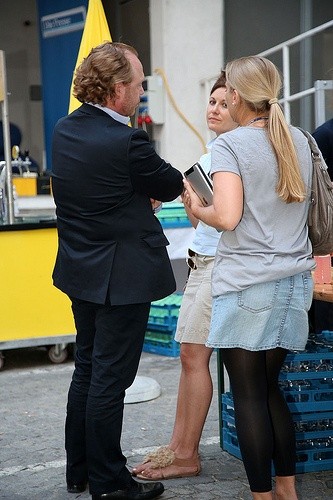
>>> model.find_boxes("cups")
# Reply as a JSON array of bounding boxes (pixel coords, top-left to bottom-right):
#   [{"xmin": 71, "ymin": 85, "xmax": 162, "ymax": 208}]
[{"xmin": 314, "ymin": 256, "xmax": 333, "ymax": 292}]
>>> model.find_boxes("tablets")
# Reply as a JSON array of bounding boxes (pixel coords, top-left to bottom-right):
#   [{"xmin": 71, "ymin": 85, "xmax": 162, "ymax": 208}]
[{"xmin": 184, "ymin": 162, "xmax": 224, "ymax": 233}]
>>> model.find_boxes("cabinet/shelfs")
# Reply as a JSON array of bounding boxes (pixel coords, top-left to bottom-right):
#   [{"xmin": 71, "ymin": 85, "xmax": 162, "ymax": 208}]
[{"xmin": 0, "ymin": 193, "xmax": 81, "ymax": 350}]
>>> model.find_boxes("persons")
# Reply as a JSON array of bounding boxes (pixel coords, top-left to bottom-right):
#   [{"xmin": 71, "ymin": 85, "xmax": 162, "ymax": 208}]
[
  {"xmin": 130, "ymin": 69, "xmax": 238, "ymax": 481},
  {"xmin": 48, "ymin": 40, "xmax": 182, "ymax": 500},
  {"xmin": 181, "ymin": 55, "xmax": 329, "ymax": 500},
  {"xmin": 0, "ymin": 119, "xmax": 22, "ymax": 161},
  {"xmin": 311, "ymin": 118, "xmax": 333, "ymax": 182}
]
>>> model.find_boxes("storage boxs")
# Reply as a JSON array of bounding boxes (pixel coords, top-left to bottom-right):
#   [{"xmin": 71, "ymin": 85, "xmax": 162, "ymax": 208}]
[
  {"xmin": 142, "ymin": 290, "xmax": 185, "ymax": 358},
  {"xmin": 217, "ymin": 331, "xmax": 333, "ymax": 473},
  {"xmin": 12, "ymin": 174, "xmax": 37, "ymax": 197}
]
